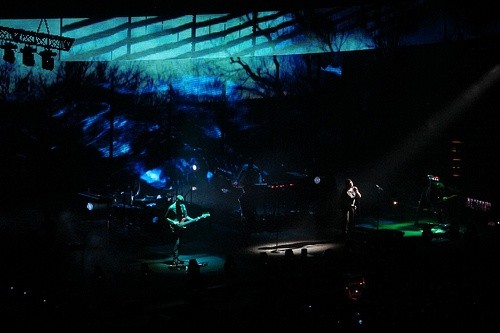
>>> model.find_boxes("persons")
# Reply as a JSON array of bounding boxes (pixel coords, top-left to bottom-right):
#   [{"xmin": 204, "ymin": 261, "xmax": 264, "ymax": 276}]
[
  {"xmin": 163, "ymin": 194, "xmax": 199, "ymax": 266},
  {"xmin": 130, "ymin": 178, "xmax": 146, "ymax": 227},
  {"xmin": 426, "ymin": 176, "xmax": 448, "ymax": 225},
  {"xmin": 237, "ymin": 184, "xmax": 257, "ymax": 244},
  {"xmin": 341, "ymin": 178, "xmax": 362, "ymax": 241}
]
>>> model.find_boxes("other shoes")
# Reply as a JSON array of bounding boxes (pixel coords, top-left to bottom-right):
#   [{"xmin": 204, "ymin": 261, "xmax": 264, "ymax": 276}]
[{"xmin": 172, "ymin": 258, "xmax": 184, "ymax": 264}]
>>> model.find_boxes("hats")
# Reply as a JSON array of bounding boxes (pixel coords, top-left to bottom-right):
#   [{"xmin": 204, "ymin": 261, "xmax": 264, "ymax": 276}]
[{"xmin": 176, "ymin": 194, "xmax": 184, "ymax": 203}]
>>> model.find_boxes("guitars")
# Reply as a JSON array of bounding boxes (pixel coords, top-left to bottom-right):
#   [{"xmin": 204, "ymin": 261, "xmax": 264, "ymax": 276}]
[{"xmin": 170, "ymin": 212, "xmax": 211, "ymax": 232}]
